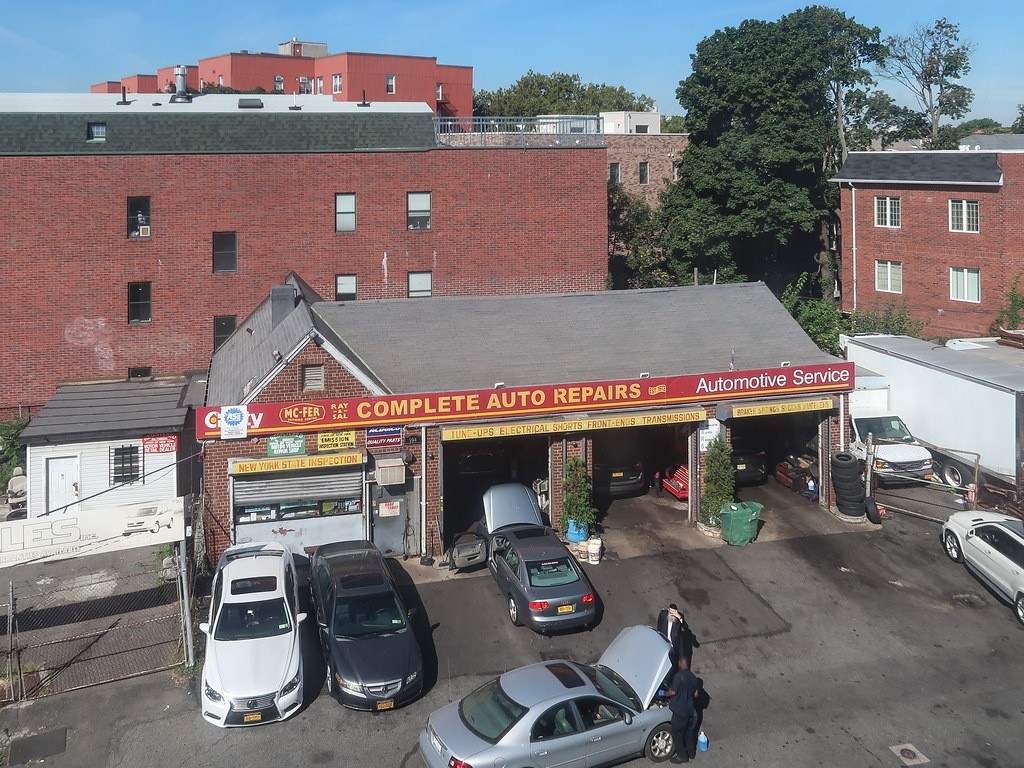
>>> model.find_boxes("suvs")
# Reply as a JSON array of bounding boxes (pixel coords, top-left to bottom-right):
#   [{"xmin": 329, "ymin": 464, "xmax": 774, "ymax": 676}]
[{"xmin": 848, "ymin": 410, "xmax": 934, "ymax": 489}]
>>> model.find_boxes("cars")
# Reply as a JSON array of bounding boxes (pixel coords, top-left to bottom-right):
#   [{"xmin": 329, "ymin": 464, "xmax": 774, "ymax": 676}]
[
  {"xmin": 307, "ymin": 539, "xmax": 441, "ymax": 712},
  {"xmin": 198, "ymin": 540, "xmax": 307, "ymax": 729},
  {"xmin": 418, "ymin": 626, "xmax": 674, "ymax": 768},
  {"xmin": 731, "ymin": 448, "xmax": 768, "ymax": 488},
  {"xmin": 593, "ymin": 446, "xmax": 650, "ymax": 499},
  {"xmin": 449, "ymin": 482, "xmax": 596, "ymax": 635},
  {"xmin": 942, "ymin": 510, "xmax": 1024, "ymax": 626}
]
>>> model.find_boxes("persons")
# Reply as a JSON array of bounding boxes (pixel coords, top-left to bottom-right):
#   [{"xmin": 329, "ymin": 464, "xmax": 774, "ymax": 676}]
[
  {"xmin": 652, "ymin": 463, "xmax": 679, "ymax": 498},
  {"xmin": 657, "ymin": 604, "xmax": 688, "ymax": 657},
  {"xmin": 664, "ymin": 657, "xmax": 700, "ymax": 763}
]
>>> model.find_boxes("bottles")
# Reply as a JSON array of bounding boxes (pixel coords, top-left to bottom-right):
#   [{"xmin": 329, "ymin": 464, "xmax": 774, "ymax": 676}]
[{"xmin": 698, "ymin": 731, "xmax": 708, "ymax": 752}]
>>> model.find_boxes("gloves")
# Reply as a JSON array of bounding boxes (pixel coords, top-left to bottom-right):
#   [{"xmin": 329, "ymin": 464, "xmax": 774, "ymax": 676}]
[{"xmin": 658, "ymin": 690, "xmax": 665, "ymax": 696}]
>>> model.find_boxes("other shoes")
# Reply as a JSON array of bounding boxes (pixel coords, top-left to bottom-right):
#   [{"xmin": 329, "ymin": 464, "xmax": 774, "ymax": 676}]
[
  {"xmin": 687, "ymin": 752, "xmax": 696, "ymax": 760},
  {"xmin": 670, "ymin": 755, "xmax": 688, "ymax": 764}
]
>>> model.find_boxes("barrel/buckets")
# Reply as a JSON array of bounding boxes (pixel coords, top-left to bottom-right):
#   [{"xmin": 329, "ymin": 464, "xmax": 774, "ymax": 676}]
[{"xmin": 577, "ymin": 539, "xmax": 603, "ymax": 564}]
